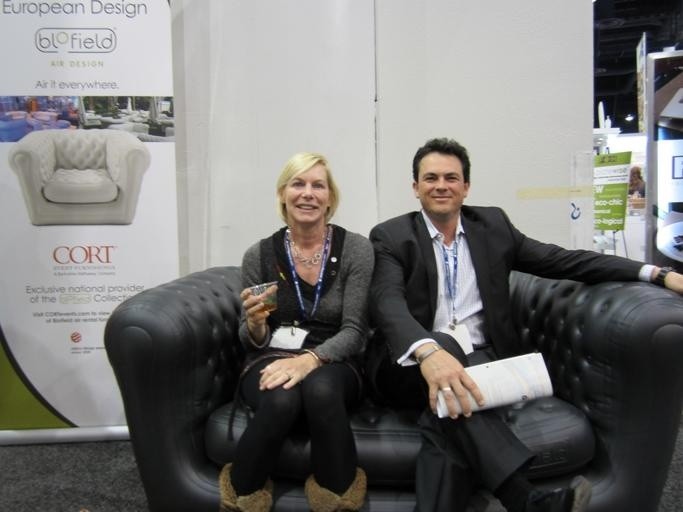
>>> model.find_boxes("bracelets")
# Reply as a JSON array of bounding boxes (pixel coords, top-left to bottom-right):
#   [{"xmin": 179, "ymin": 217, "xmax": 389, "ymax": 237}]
[
  {"xmin": 652, "ymin": 265, "xmax": 678, "ymax": 288},
  {"xmin": 299, "ymin": 344, "xmax": 322, "ymax": 370},
  {"xmin": 415, "ymin": 344, "xmax": 444, "ymax": 365}
]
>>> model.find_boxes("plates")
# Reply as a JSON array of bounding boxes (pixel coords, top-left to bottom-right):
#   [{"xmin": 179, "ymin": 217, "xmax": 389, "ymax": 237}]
[{"xmin": 656, "ymin": 219, "xmax": 682, "ymax": 262}]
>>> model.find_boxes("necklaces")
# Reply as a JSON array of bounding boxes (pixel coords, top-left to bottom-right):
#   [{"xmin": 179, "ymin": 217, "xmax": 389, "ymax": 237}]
[{"xmin": 283, "ymin": 222, "xmax": 329, "ymax": 270}]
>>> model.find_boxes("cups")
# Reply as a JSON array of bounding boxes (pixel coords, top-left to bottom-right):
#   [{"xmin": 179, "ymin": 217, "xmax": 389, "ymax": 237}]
[{"xmin": 251, "ymin": 280, "xmax": 280, "ymax": 313}]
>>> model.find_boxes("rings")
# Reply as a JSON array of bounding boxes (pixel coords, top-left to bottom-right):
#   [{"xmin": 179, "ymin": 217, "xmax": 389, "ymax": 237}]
[
  {"xmin": 263, "ymin": 364, "xmax": 273, "ymax": 375},
  {"xmin": 284, "ymin": 369, "xmax": 295, "ymax": 383},
  {"xmin": 442, "ymin": 385, "xmax": 452, "ymax": 397}
]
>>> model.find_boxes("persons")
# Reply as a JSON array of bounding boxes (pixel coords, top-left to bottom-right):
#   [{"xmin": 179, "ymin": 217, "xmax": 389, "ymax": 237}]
[
  {"xmin": 365, "ymin": 136, "xmax": 682, "ymax": 511},
  {"xmin": 217, "ymin": 149, "xmax": 379, "ymax": 511},
  {"xmin": 627, "ymin": 164, "xmax": 645, "ymax": 199}
]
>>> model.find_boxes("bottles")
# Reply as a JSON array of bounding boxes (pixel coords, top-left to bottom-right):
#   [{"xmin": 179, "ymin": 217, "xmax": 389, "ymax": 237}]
[{"xmin": 604, "ymin": 115, "xmax": 612, "ymax": 128}]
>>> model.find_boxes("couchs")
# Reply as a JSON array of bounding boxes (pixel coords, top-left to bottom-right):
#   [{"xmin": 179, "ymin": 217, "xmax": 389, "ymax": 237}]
[
  {"xmin": 7, "ymin": 125, "xmax": 150, "ymax": 227},
  {"xmin": 100, "ymin": 256, "xmax": 682, "ymax": 512}
]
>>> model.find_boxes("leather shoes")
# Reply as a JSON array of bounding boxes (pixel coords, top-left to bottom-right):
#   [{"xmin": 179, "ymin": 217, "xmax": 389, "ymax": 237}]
[{"xmin": 528, "ymin": 473, "xmax": 594, "ymax": 511}]
